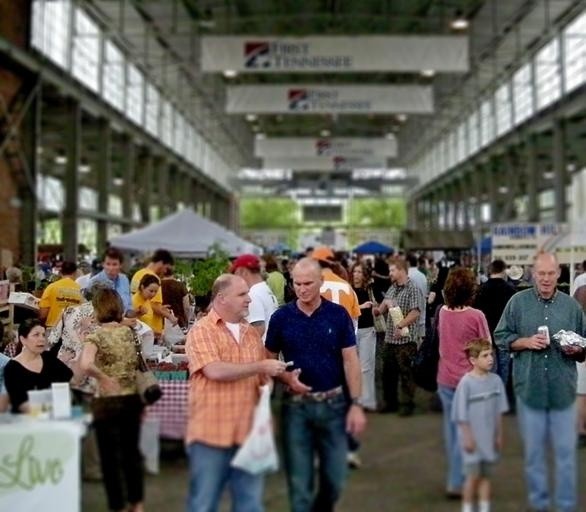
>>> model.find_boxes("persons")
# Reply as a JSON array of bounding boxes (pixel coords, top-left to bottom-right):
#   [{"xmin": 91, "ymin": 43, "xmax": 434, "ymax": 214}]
[
  {"xmin": 161, "ymin": 266, "xmax": 191, "ymax": 331},
  {"xmin": 307, "ymin": 245, "xmax": 361, "ymax": 323},
  {"xmin": 131, "ymin": 273, "xmax": 159, "ymax": 327},
  {"xmin": 226, "ymin": 253, "xmax": 278, "ymax": 346},
  {"xmin": 372, "ymin": 255, "xmax": 425, "ymax": 418},
  {"xmin": 428, "ymin": 267, "xmax": 449, "ymax": 327},
  {"xmin": 493, "ymin": 253, "xmax": 586, "ymax": 512},
  {"xmin": 473, "ymin": 258, "xmax": 517, "ymax": 416},
  {"xmin": 47, "ymin": 283, "xmax": 112, "ymax": 403},
  {"xmin": 89, "ymin": 248, "xmax": 147, "ymax": 319},
  {"xmin": 185, "ymin": 274, "xmax": 286, "ymax": 510},
  {"xmin": 0, "ymin": 321, "xmax": 12, "ymax": 413},
  {"xmin": 266, "ymin": 261, "xmax": 287, "ymax": 307},
  {"xmin": 406, "ymin": 254, "xmax": 427, "ymax": 348},
  {"xmin": 264, "ymin": 257, "xmax": 366, "ymax": 512},
  {"xmin": 436, "ymin": 269, "xmax": 492, "ymax": 500},
  {"xmin": 349, "ymin": 261, "xmax": 378, "ymax": 414},
  {"xmin": 129, "ymin": 248, "xmax": 180, "ymax": 344},
  {"xmin": 80, "ymin": 287, "xmax": 145, "ymax": 512},
  {"xmin": 5, "ymin": 260, "xmax": 26, "ymax": 298},
  {"xmin": 450, "ymin": 338, "xmax": 509, "ymax": 512},
  {"xmin": 39, "ymin": 261, "xmax": 83, "ymax": 327},
  {"xmin": 573, "ymin": 260, "xmax": 586, "ymax": 295},
  {"xmin": 573, "ymin": 287, "xmax": 586, "ymax": 450},
  {"xmin": 4, "ymin": 318, "xmax": 76, "ymax": 415}
]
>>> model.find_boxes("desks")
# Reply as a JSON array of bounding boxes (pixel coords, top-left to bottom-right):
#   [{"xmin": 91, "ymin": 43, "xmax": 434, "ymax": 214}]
[
  {"xmin": 144, "ymin": 379, "xmax": 190, "ymax": 440},
  {"xmin": 0, "ymin": 404, "xmax": 93, "ymax": 511}
]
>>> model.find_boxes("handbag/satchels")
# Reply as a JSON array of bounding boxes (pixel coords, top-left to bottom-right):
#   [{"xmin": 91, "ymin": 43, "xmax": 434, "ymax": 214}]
[{"xmin": 128, "ymin": 325, "xmax": 164, "ymax": 407}]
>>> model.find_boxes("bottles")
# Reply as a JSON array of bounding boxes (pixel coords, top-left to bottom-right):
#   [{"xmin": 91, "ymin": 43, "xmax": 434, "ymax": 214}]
[{"xmin": 539, "ymin": 325, "xmax": 551, "ymax": 345}]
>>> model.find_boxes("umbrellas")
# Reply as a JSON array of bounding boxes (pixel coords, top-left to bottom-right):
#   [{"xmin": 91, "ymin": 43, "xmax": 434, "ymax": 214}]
[
  {"xmin": 352, "ymin": 241, "xmax": 395, "ymax": 255},
  {"xmin": 110, "ymin": 209, "xmax": 263, "ymax": 260}
]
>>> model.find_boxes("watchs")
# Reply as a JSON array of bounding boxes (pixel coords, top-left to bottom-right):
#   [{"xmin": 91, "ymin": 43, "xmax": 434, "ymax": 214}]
[{"xmin": 350, "ymin": 397, "xmax": 365, "ymax": 408}]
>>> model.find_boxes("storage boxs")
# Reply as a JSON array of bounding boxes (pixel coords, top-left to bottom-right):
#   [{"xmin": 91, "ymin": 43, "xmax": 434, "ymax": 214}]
[
  {"xmin": 171, "ymin": 345, "xmax": 185, "ymax": 353},
  {"xmin": 8, "ymin": 292, "xmax": 40, "ymax": 310},
  {"xmin": 171, "ymin": 353, "xmax": 187, "ymax": 366},
  {"xmin": 171, "ymin": 371, "xmax": 187, "ymax": 380},
  {"xmin": 155, "ymin": 371, "xmax": 170, "ymax": 380},
  {"xmin": 174, "ymin": 335, "xmax": 186, "ymax": 345}
]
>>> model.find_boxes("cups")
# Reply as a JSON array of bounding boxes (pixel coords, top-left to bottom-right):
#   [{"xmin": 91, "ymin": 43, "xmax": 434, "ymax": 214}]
[
  {"xmin": 52, "ymin": 383, "xmax": 70, "ymax": 418},
  {"xmin": 27, "ymin": 390, "xmax": 45, "ymax": 417}
]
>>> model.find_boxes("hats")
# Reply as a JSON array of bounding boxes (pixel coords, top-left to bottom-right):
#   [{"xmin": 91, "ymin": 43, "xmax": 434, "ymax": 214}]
[
  {"xmin": 505, "ymin": 266, "xmax": 523, "ymax": 279},
  {"xmin": 312, "ymin": 247, "xmax": 335, "ymax": 263},
  {"xmin": 230, "ymin": 255, "xmax": 260, "ymax": 272}
]
